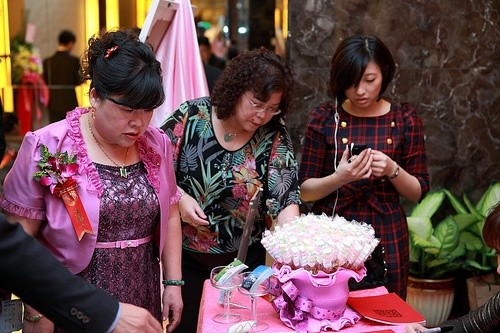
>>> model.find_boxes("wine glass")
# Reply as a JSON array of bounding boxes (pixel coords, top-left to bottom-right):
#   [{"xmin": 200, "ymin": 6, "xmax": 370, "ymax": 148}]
[
  {"xmin": 209, "ymin": 265, "xmax": 243, "ymax": 323},
  {"xmin": 234, "ymin": 272, "xmax": 271, "ymax": 332}
]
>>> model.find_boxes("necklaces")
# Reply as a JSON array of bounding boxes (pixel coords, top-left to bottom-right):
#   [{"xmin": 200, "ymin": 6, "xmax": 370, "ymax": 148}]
[
  {"xmin": 86, "ymin": 111, "xmax": 131, "ymax": 179},
  {"xmin": 219, "ymin": 117, "xmax": 248, "ymax": 142}
]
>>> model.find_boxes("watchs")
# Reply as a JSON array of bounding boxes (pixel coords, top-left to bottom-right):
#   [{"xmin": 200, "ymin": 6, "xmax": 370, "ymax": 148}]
[{"xmin": 388, "ymin": 164, "xmax": 401, "ymax": 180}]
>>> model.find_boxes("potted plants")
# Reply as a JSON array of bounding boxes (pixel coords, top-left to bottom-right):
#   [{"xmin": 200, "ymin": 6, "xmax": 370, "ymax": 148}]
[{"xmin": 406, "ymin": 183, "xmax": 500, "ymax": 328}]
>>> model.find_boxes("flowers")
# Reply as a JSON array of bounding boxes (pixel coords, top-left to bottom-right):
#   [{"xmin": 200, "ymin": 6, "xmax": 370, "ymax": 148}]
[{"xmin": 32, "ymin": 144, "xmax": 80, "ymax": 195}]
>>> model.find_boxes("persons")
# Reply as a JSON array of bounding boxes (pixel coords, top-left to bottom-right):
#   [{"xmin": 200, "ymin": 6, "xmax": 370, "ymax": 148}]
[
  {"xmin": 42, "ymin": 28, "xmax": 86, "ymax": 126},
  {"xmin": 0, "ymin": 210, "xmax": 164, "ymax": 333},
  {"xmin": 196, "ymin": 36, "xmax": 227, "ymax": 97},
  {"xmin": 157, "ymin": 49, "xmax": 301, "ymax": 333},
  {"xmin": 0, "ymin": 28, "xmax": 186, "ymax": 333},
  {"xmin": 405, "ymin": 202, "xmax": 500, "ymax": 333},
  {"xmin": 299, "ymin": 34, "xmax": 430, "ymax": 308}
]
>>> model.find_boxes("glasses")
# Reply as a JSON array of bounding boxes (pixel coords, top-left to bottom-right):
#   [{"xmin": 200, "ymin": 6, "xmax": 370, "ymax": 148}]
[{"xmin": 244, "ymin": 89, "xmax": 281, "ymax": 115}]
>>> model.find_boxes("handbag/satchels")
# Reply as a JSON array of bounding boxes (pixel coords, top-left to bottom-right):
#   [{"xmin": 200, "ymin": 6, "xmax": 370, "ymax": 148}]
[{"xmin": 349, "ymin": 240, "xmax": 388, "ymax": 290}]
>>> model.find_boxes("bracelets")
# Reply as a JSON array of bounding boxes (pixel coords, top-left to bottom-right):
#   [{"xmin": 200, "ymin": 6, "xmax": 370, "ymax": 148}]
[
  {"xmin": 162, "ymin": 279, "xmax": 185, "ymax": 286},
  {"xmin": 24, "ymin": 312, "xmax": 44, "ymax": 321}
]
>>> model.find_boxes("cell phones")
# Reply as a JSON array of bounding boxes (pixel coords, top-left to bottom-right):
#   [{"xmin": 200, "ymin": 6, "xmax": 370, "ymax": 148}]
[{"xmin": 348, "ymin": 144, "xmax": 368, "ymax": 156}]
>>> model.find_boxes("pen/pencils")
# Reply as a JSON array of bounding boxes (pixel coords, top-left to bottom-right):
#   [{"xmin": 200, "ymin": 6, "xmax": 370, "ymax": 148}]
[{"xmin": 414, "ymin": 326, "xmax": 455, "ymax": 333}]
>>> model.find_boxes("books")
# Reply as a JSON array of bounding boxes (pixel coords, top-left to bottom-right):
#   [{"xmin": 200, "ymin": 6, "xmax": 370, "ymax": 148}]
[{"xmin": 346, "ymin": 293, "xmax": 427, "ymax": 327}]
